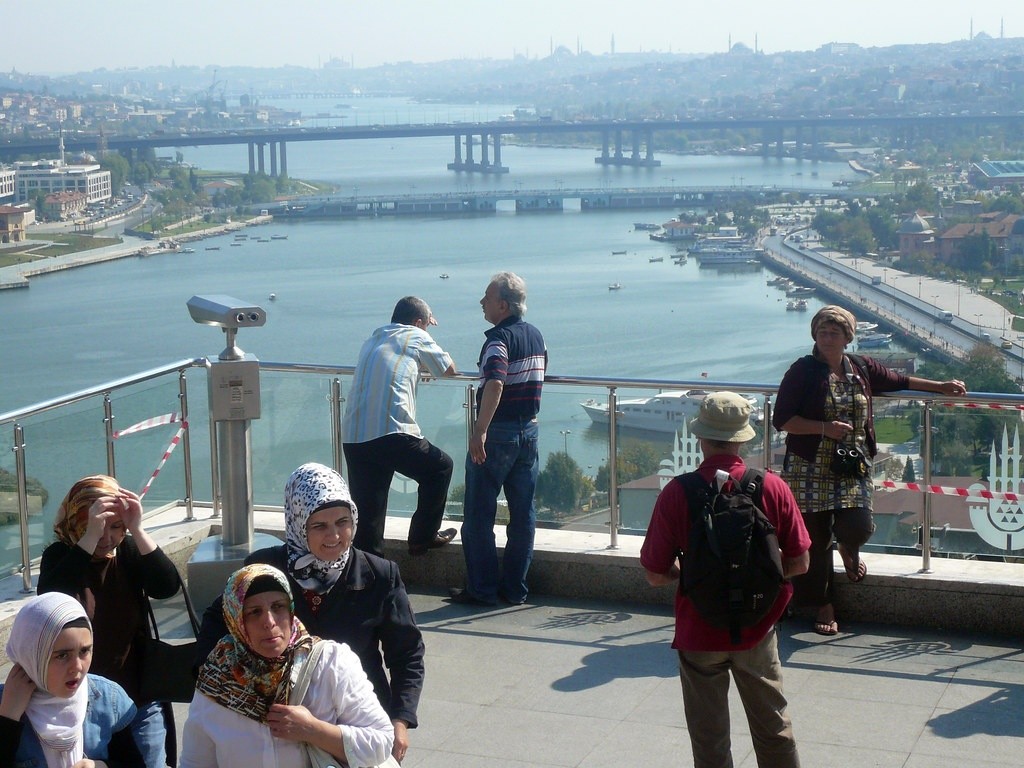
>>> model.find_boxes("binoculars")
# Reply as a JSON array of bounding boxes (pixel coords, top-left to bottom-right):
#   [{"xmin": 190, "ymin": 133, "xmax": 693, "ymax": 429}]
[
  {"xmin": 831, "ymin": 448, "xmax": 858, "ymax": 474},
  {"xmin": 187, "ymin": 294, "xmax": 267, "ymax": 328}
]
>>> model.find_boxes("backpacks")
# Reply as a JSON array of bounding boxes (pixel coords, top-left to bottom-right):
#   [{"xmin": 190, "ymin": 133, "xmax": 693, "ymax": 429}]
[{"xmin": 673, "ymin": 468, "xmax": 788, "ymax": 632}]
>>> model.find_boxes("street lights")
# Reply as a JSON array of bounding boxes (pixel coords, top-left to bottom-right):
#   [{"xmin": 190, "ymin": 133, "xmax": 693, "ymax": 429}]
[{"xmin": 559, "ymin": 430, "xmax": 571, "ymax": 459}]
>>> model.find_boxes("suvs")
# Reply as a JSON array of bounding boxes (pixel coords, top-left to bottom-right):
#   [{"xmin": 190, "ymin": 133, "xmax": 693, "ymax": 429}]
[{"xmin": 984, "ymin": 334, "xmax": 990, "ymax": 340}]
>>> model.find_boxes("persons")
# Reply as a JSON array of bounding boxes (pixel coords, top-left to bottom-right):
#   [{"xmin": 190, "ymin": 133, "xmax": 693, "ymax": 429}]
[
  {"xmin": 451, "ymin": 272, "xmax": 548, "ymax": 605},
  {"xmin": 37, "ymin": 474, "xmax": 181, "ymax": 768},
  {"xmin": 640, "ymin": 390, "xmax": 812, "ymax": 768},
  {"xmin": 200, "ymin": 462, "xmax": 425, "ymax": 766},
  {"xmin": 178, "ymin": 564, "xmax": 395, "ymax": 768},
  {"xmin": 0, "ymin": 592, "xmax": 148, "ymax": 768},
  {"xmin": 773, "ymin": 304, "xmax": 966, "ymax": 635},
  {"xmin": 341, "ymin": 296, "xmax": 457, "ymax": 557}
]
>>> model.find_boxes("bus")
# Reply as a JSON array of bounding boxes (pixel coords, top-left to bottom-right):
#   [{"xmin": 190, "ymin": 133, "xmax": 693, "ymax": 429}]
[{"xmin": 770, "ymin": 226, "xmax": 777, "ymax": 236}]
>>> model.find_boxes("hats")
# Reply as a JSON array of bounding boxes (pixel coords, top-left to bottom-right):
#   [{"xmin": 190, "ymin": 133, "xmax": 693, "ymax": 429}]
[{"xmin": 690, "ymin": 391, "xmax": 756, "ymax": 443}]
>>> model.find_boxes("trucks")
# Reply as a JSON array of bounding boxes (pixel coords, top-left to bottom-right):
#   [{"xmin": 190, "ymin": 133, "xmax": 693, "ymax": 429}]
[{"xmin": 872, "ymin": 276, "xmax": 881, "ymax": 284}]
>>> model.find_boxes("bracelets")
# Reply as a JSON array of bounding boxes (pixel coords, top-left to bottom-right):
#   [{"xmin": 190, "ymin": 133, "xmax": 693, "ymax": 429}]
[{"xmin": 821, "ymin": 421, "xmax": 825, "ymax": 439}]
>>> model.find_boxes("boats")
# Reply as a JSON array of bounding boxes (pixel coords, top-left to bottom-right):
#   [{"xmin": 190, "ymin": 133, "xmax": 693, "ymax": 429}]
[
  {"xmin": 857, "ymin": 330, "xmax": 892, "ymax": 347},
  {"xmin": 608, "ymin": 282, "xmax": 620, "ymax": 289},
  {"xmin": 634, "ymin": 223, "xmax": 661, "ymax": 229},
  {"xmin": 271, "ymin": 235, "xmax": 288, "ymax": 239},
  {"xmin": 767, "ymin": 276, "xmax": 817, "ymax": 297},
  {"xmin": 649, "ymin": 256, "xmax": 663, "ymax": 261},
  {"xmin": 855, "ymin": 322, "xmax": 878, "ymax": 331},
  {"xmin": 612, "ymin": 250, "xmax": 627, "ymax": 254},
  {"xmin": 786, "ymin": 298, "xmax": 808, "ymax": 310},
  {"xmin": 579, "ymin": 373, "xmax": 774, "ymax": 436},
  {"xmin": 670, "ymin": 254, "xmax": 687, "ymax": 264},
  {"xmin": 688, "ymin": 233, "xmax": 760, "ymax": 263}
]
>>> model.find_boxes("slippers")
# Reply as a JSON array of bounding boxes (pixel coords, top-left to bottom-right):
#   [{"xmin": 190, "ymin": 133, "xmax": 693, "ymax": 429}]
[
  {"xmin": 840, "ymin": 546, "xmax": 867, "ymax": 584},
  {"xmin": 814, "ymin": 600, "xmax": 839, "ymax": 636}
]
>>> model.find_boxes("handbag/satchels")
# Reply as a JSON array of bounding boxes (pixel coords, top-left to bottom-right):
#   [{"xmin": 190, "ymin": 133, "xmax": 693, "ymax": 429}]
[
  {"xmin": 133, "ymin": 578, "xmax": 202, "ymax": 703},
  {"xmin": 307, "ymin": 747, "xmax": 404, "ymax": 768}
]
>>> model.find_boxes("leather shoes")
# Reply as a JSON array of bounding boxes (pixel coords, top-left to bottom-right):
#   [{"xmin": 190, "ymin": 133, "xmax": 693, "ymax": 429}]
[{"xmin": 408, "ymin": 527, "xmax": 458, "ymax": 555}]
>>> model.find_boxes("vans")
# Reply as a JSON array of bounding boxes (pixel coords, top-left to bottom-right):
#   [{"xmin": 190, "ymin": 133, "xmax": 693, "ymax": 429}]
[{"xmin": 939, "ymin": 311, "xmax": 952, "ymax": 321}]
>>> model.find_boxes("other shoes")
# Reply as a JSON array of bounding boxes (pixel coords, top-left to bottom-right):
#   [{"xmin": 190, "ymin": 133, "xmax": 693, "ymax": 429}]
[
  {"xmin": 448, "ymin": 587, "xmax": 495, "ymax": 606},
  {"xmin": 498, "ymin": 590, "xmax": 522, "ymax": 605}
]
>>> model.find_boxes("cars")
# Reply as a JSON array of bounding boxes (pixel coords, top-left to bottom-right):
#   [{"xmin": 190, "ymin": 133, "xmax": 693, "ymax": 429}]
[{"xmin": 1002, "ymin": 341, "xmax": 1012, "ymax": 348}]
